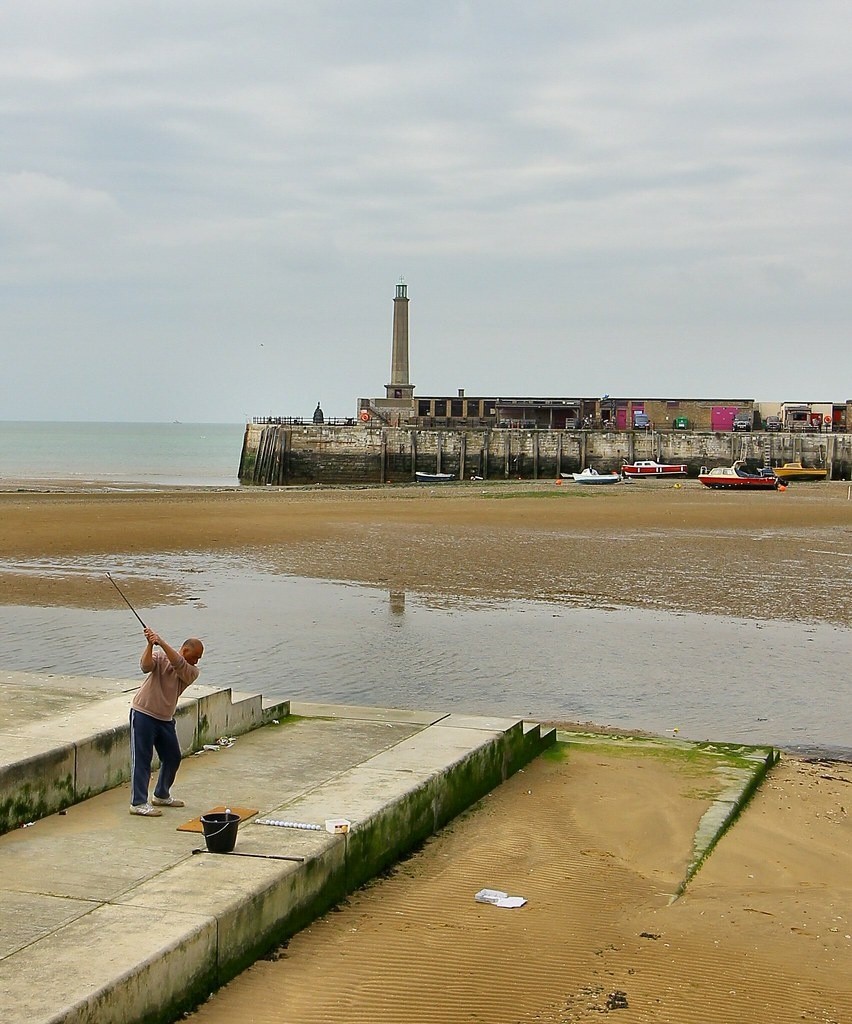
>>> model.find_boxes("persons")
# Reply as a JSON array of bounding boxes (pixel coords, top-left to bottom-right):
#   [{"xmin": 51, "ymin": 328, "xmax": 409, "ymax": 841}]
[
  {"xmin": 510, "ymin": 421, "xmax": 514, "ymax": 427},
  {"xmin": 516, "ymin": 420, "xmax": 521, "ymax": 428},
  {"xmin": 129, "ymin": 627, "xmax": 203, "ymax": 817},
  {"xmin": 524, "ymin": 421, "xmax": 531, "ymax": 428}
]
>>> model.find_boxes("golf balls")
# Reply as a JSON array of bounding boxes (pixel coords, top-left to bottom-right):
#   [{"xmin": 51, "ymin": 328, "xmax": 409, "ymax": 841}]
[
  {"xmin": 266, "ymin": 820, "xmax": 322, "ymax": 830},
  {"xmin": 225, "ymin": 808, "xmax": 231, "ymax": 813},
  {"xmin": 255, "ymin": 819, "xmax": 260, "ymax": 823}
]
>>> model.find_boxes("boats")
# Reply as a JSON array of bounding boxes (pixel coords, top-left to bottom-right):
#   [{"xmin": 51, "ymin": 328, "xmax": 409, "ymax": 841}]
[
  {"xmin": 757, "ymin": 463, "xmax": 828, "ymax": 481},
  {"xmin": 622, "ymin": 461, "xmax": 688, "ymax": 479},
  {"xmin": 698, "ymin": 459, "xmax": 789, "ymax": 490},
  {"xmin": 415, "ymin": 471, "xmax": 455, "ymax": 483},
  {"xmin": 572, "ymin": 472, "xmax": 622, "ymax": 485},
  {"xmin": 559, "ymin": 469, "xmax": 598, "ymax": 479}
]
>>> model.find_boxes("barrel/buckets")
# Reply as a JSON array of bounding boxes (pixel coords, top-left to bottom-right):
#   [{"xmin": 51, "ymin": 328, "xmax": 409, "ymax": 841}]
[{"xmin": 200, "ymin": 813, "xmax": 241, "ymax": 853}]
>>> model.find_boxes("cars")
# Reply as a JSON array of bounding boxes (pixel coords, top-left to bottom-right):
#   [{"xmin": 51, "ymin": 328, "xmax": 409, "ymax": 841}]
[
  {"xmin": 764, "ymin": 416, "xmax": 782, "ymax": 432},
  {"xmin": 732, "ymin": 414, "xmax": 752, "ymax": 432},
  {"xmin": 632, "ymin": 414, "xmax": 651, "ymax": 430}
]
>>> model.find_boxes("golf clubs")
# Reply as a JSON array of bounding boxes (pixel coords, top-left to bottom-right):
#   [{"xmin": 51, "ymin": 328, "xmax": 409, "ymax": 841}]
[
  {"xmin": 104, "ymin": 571, "xmax": 158, "ymax": 645},
  {"xmin": 191, "ymin": 848, "xmax": 305, "ymax": 862}
]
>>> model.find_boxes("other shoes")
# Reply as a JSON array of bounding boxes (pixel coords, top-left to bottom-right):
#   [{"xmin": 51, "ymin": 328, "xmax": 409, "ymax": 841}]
[
  {"xmin": 129, "ymin": 803, "xmax": 162, "ymax": 816},
  {"xmin": 151, "ymin": 795, "xmax": 184, "ymax": 806}
]
